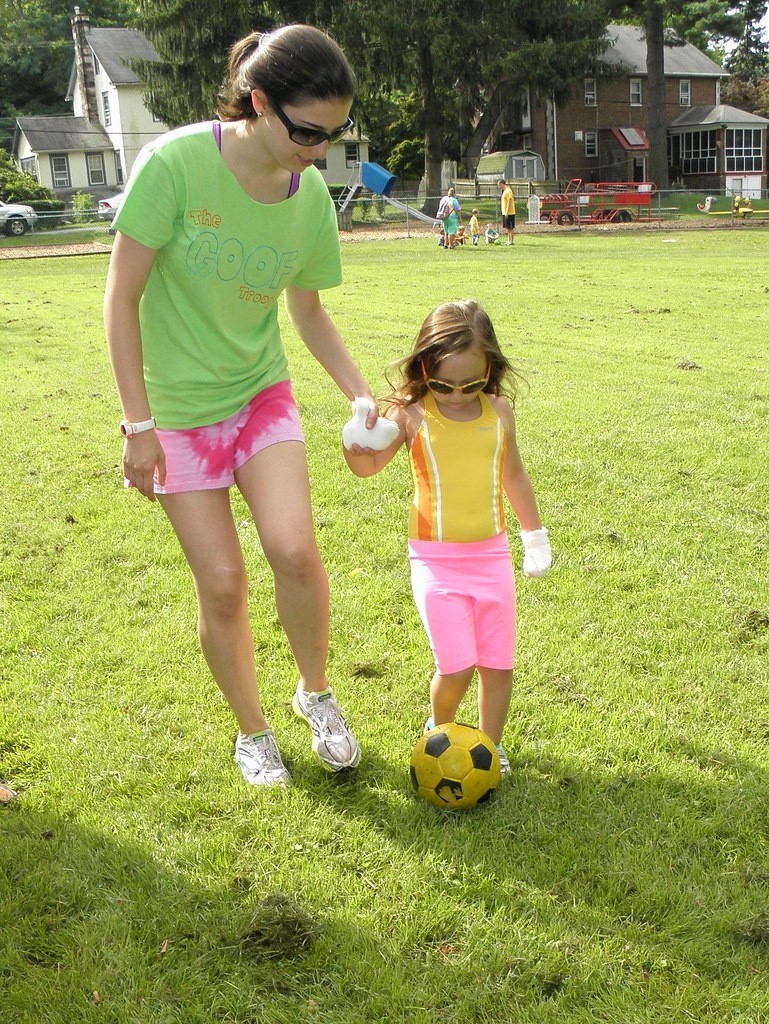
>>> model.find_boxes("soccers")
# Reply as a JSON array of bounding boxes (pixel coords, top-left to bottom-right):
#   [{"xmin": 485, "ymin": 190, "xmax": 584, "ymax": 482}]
[{"xmin": 409, "ymin": 723, "xmax": 501, "ymax": 811}]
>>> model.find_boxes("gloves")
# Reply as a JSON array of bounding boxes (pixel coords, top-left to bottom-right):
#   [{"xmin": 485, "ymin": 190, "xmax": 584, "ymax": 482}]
[
  {"xmin": 521, "ymin": 527, "xmax": 553, "ymax": 579},
  {"xmin": 342, "ymin": 397, "xmax": 400, "ymax": 458}
]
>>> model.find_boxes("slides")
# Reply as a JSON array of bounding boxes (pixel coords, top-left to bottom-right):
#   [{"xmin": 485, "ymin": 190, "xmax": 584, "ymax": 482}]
[{"xmin": 362, "ymin": 162, "xmax": 443, "ymax": 228}]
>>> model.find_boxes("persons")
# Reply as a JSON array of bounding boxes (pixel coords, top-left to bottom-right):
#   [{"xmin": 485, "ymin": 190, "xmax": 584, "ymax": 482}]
[
  {"xmin": 342, "ymin": 301, "xmax": 551, "ymax": 781},
  {"xmin": 438, "ymin": 228, "xmax": 445, "ymax": 245},
  {"xmin": 496, "ymin": 179, "xmax": 515, "ymax": 246},
  {"xmin": 485, "ymin": 223, "xmax": 499, "ymax": 245},
  {"xmin": 455, "ymin": 226, "xmax": 468, "ymax": 246},
  {"xmin": 469, "ymin": 209, "xmax": 480, "ymax": 246},
  {"xmin": 103, "ymin": 22, "xmax": 380, "ymax": 787},
  {"xmin": 441, "ymin": 188, "xmax": 462, "ymax": 249}
]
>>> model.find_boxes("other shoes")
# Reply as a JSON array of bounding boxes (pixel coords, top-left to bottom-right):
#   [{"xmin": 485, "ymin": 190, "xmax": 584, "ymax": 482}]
[
  {"xmin": 505, "ymin": 242, "xmax": 513, "ymax": 246},
  {"xmin": 449, "ymin": 246, "xmax": 455, "ymax": 249},
  {"xmin": 443, "ymin": 245, "xmax": 448, "ymax": 249},
  {"xmin": 474, "ymin": 242, "xmax": 477, "ymax": 246}
]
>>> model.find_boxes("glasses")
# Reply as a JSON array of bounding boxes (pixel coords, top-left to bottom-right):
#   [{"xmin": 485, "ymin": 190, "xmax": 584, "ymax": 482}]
[
  {"xmin": 420, "ymin": 360, "xmax": 491, "ymax": 397},
  {"xmin": 268, "ymin": 96, "xmax": 355, "ymax": 148}
]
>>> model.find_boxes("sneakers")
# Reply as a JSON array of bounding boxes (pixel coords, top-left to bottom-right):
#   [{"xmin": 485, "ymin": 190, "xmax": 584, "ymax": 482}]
[
  {"xmin": 290, "ymin": 677, "xmax": 363, "ymax": 774},
  {"xmin": 234, "ymin": 726, "xmax": 293, "ymax": 794}
]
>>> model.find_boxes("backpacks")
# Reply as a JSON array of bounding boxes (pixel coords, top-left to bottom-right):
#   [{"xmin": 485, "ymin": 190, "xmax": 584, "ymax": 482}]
[{"xmin": 435, "ymin": 198, "xmax": 453, "ymax": 220}]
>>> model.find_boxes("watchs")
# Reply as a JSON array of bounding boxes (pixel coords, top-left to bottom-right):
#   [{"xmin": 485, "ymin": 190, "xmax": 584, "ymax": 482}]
[{"xmin": 120, "ymin": 418, "xmax": 158, "ymax": 440}]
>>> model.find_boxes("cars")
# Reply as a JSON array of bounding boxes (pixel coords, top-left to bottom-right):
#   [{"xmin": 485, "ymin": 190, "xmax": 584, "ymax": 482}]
[
  {"xmin": 0, "ymin": 200, "xmax": 39, "ymax": 237},
  {"xmin": 98, "ymin": 193, "xmax": 124, "ymax": 223}
]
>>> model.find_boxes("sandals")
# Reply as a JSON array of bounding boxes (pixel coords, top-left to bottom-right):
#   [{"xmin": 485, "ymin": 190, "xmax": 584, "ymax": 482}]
[
  {"xmin": 494, "ymin": 746, "xmax": 510, "ymax": 781},
  {"xmin": 424, "ymin": 716, "xmax": 438, "ymax": 738}
]
